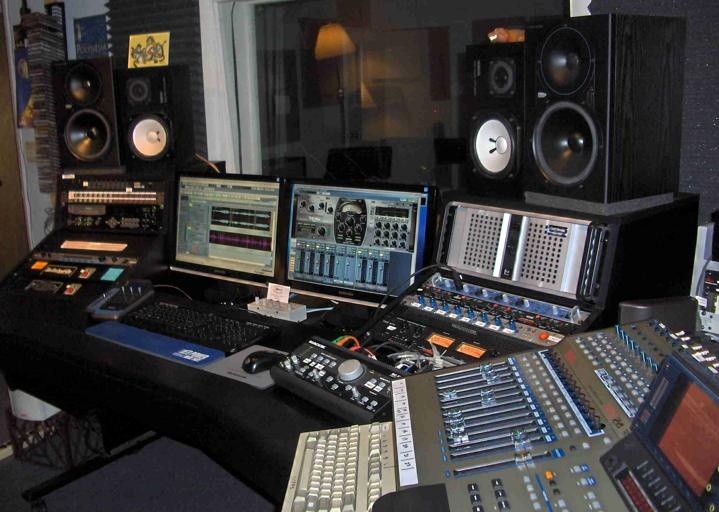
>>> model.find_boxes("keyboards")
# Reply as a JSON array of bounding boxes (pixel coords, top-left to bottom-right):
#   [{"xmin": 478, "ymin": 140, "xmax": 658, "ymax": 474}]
[
  {"xmin": 281, "ymin": 421, "xmax": 398, "ymax": 512},
  {"xmin": 120, "ymin": 292, "xmax": 276, "ymax": 354}
]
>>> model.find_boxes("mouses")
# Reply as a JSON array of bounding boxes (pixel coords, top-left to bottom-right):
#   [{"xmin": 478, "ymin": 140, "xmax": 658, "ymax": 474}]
[{"xmin": 242, "ymin": 351, "xmax": 287, "ymax": 374}]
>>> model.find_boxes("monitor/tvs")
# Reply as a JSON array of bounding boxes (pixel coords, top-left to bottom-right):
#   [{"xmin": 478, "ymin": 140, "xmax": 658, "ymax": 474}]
[
  {"xmin": 285, "ymin": 178, "xmax": 439, "ymax": 328},
  {"xmin": 170, "ymin": 170, "xmax": 286, "ymax": 301}
]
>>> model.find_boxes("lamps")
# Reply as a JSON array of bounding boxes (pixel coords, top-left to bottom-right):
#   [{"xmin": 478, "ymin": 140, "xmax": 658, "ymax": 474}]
[{"xmin": 315, "ymin": 21, "xmax": 357, "ymax": 146}]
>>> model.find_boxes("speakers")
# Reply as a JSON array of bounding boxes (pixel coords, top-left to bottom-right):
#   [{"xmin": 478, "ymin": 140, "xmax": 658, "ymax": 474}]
[
  {"xmin": 524, "ymin": 14, "xmax": 687, "ymax": 217},
  {"xmin": 50, "ymin": 57, "xmax": 121, "ymax": 170},
  {"xmin": 459, "ymin": 43, "xmax": 526, "ymax": 200},
  {"xmin": 115, "ymin": 63, "xmax": 194, "ymax": 172}
]
